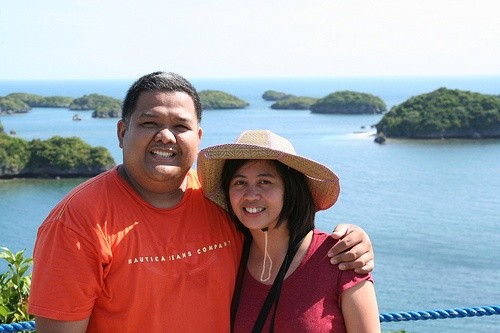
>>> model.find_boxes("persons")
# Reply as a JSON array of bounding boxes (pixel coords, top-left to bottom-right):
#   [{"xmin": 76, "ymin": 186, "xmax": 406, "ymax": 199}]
[
  {"xmin": 28, "ymin": 71, "xmax": 375, "ymax": 333},
  {"xmin": 197, "ymin": 131, "xmax": 383, "ymax": 333}
]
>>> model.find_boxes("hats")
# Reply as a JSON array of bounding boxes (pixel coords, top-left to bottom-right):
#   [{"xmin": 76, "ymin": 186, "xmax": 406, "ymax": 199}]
[{"xmin": 196, "ymin": 130, "xmax": 340, "ymax": 213}]
